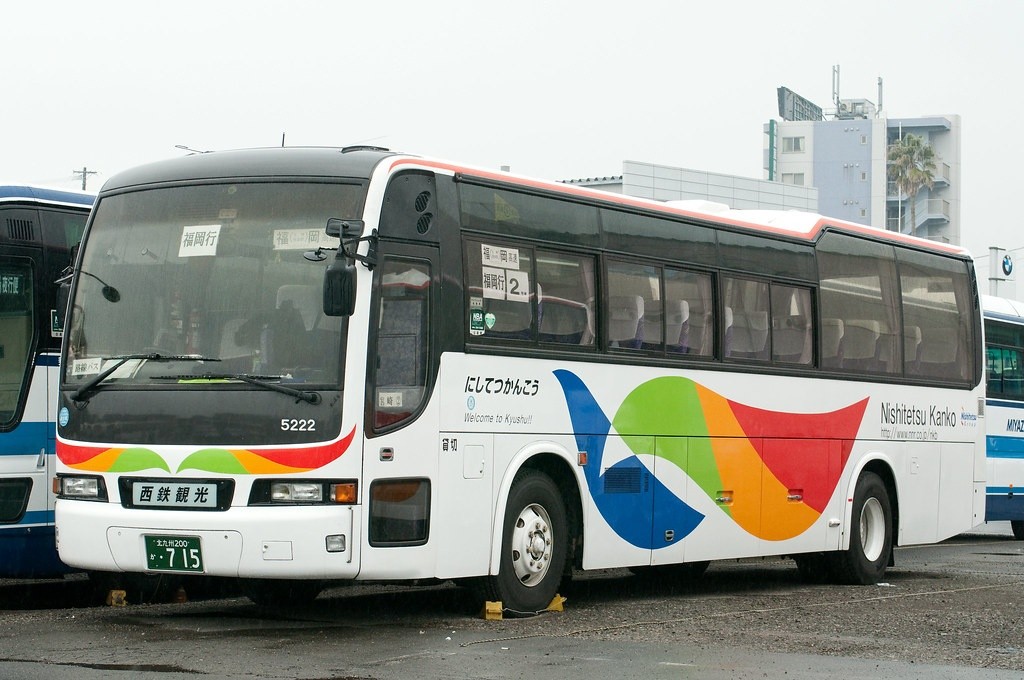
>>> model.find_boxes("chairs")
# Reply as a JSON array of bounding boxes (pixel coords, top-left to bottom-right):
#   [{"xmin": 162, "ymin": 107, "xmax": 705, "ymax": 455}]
[{"xmin": 215, "ymin": 281, "xmax": 961, "ymax": 376}]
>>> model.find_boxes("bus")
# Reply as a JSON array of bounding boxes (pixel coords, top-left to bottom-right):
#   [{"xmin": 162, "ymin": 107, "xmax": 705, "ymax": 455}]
[
  {"xmin": 0, "ymin": 145, "xmax": 986, "ymax": 617},
  {"xmin": 820, "ymin": 280, "xmax": 1024, "ymax": 540}
]
